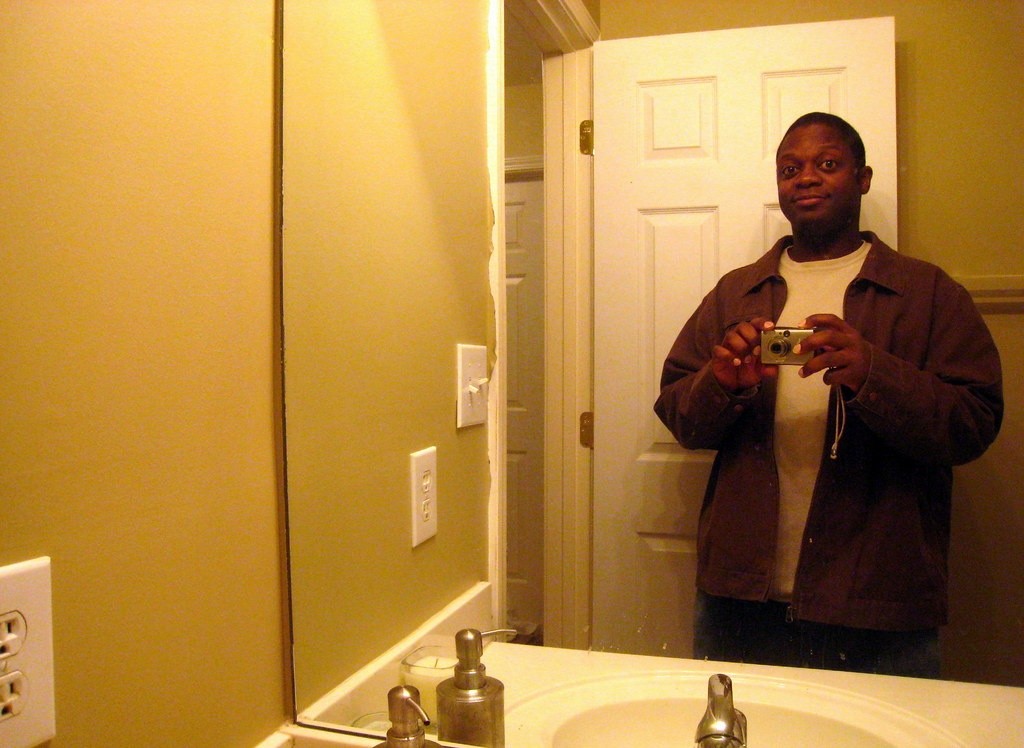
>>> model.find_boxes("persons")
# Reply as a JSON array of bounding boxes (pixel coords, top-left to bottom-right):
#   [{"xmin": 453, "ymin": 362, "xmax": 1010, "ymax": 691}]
[{"xmin": 655, "ymin": 112, "xmax": 1006, "ymax": 678}]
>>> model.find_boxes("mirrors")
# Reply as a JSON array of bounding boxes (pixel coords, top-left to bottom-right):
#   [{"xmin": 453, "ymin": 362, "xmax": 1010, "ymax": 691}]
[{"xmin": 274, "ymin": 0, "xmax": 1024, "ymax": 748}]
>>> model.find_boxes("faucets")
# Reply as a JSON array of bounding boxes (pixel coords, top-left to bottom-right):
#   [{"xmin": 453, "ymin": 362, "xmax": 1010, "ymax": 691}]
[{"xmin": 694, "ymin": 674, "xmax": 748, "ymax": 748}]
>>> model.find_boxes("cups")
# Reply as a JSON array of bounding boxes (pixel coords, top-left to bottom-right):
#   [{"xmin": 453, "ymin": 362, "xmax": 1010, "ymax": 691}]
[
  {"xmin": 350, "ymin": 710, "xmax": 393, "ymax": 733},
  {"xmin": 401, "ymin": 645, "xmax": 459, "ymax": 735}
]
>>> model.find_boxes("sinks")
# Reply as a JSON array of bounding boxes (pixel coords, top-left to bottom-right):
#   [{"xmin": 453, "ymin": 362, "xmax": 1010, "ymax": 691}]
[{"xmin": 506, "ymin": 673, "xmax": 967, "ymax": 748}]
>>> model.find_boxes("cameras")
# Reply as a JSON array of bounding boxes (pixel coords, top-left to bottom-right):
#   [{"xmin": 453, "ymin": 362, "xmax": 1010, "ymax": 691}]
[{"xmin": 759, "ymin": 327, "xmax": 817, "ymax": 365}]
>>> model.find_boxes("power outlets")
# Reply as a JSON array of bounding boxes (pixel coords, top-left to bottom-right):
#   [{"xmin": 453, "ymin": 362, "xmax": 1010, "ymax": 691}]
[
  {"xmin": 0, "ymin": 553, "xmax": 58, "ymax": 748},
  {"xmin": 410, "ymin": 446, "xmax": 437, "ymax": 548}
]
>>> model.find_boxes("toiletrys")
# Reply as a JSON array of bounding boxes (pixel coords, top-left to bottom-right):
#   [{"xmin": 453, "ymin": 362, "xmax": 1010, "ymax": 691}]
[
  {"xmin": 373, "ymin": 686, "xmax": 430, "ymax": 748},
  {"xmin": 435, "ymin": 627, "xmax": 516, "ymax": 748}
]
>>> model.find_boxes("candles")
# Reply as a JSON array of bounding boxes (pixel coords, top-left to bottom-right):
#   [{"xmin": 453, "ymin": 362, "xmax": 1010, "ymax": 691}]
[
  {"xmin": 400, "ymin": 644, "xmax": 458, "ymax": 730},
  {"xmin": 353, "ymin": 713, "xmax": 394, "ymax": 731}
]
